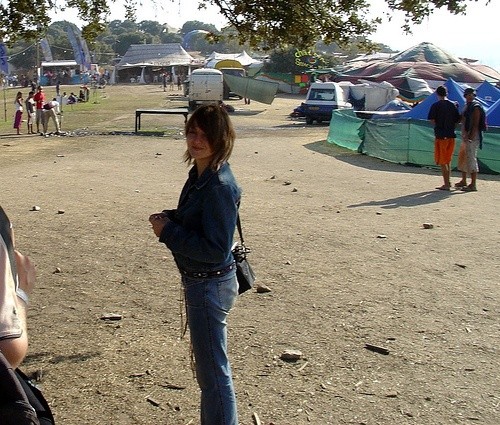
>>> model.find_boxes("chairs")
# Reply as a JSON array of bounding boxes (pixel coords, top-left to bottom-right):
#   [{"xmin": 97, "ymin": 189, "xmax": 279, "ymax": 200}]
[{"xmin": 317, "ymin": 95, "xmax": 321, "ymax": 98}]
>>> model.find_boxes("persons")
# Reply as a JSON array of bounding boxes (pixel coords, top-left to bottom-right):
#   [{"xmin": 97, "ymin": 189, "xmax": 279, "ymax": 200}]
[
  {"xmin": 427, "ymin": 86, "xmax": 459, "ymax": 191},
  {"xmin": 148, "ymin": 103, "xmax": 241, "ymax": 425},
  {"xmin": 0, "ymin": 204, "xmax": 55, "ymax": 425},
  {"xmin": 160, "ymin": 72, "xmax": 182, "ymax": 93},
  {"xmin": 0, "ymin": 68, "xmax": 90, "ymax": 134},
  {"xmin": 455, "ymin": 88, "xmax": 487, "ymax": 190}
]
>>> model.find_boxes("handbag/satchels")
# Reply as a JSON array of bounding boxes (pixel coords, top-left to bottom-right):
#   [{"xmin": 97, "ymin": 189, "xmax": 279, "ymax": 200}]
[
  {"xmin": 231, "ymin": 246, "xmax": 255, "ymax": 295},
  {"xmin": 0, "ymin": 351, "xmax": 55, "ymax": 425},
  {"xmin": 43, "ymin": 104, "xmax": 51, "ymax": 110}
]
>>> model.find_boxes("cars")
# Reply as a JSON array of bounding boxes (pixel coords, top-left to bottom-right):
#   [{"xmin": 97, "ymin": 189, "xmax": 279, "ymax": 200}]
[{"xmin": 303, "ymin": 81, "xmax": 353, "ymax": 124}]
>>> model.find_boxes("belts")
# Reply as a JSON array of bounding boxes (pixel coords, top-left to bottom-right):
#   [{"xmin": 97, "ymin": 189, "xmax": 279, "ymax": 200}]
[{"xmin": 179, "ymin": 260, "xmax": 236, "ymax": 278}]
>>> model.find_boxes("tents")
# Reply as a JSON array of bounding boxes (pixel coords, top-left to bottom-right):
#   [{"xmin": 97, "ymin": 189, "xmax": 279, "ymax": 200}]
[
  {"xmin": 205, "ymin": 50, "xmax": 266, "ymax": 80},
  {"xmin": 329, "ymin": 41, "xmax": 500, "ymax": 100},
  {"xmin": 371, "ymin": 95, "xmax": 413, "ymax": 127},
  {"xmin": 486, "ymin": 99, "xmax": 500, "ymax": 127},
  {"xmin": 472, "ymin": 79, "xmax": 500, "ymax": 106},
  {"xmin": 401, "ymin": 78, "xmax": 490, "ymax": 124},
  {"xmin": 337, "ymin": 81, "xmax": 399, "ymax": 110}
]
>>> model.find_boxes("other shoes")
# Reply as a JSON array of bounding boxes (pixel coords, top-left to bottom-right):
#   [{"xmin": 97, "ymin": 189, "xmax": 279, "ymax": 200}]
[
  {"xmin": 455, "ymin": 181, "xmax": 467, "ymax": 187},
  {"xmin": 460, "ymin": 184, "xmax": 477, "ymax": 191},
  {"xmin": 436, "ymin": 182, "xmax": 450, "ymax": 191}
]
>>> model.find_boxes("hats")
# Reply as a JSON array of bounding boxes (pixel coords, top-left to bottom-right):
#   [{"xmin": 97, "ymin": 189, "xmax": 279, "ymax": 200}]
[{"xmin": 463, "ymin": 88, "xmax": 476, "ymax": 97}]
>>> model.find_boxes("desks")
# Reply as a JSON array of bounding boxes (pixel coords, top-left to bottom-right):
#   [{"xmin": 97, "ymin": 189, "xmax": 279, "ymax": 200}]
[{"xmin": 135, "ymin": 109, "xmax": 189, "ymax": 134}]
